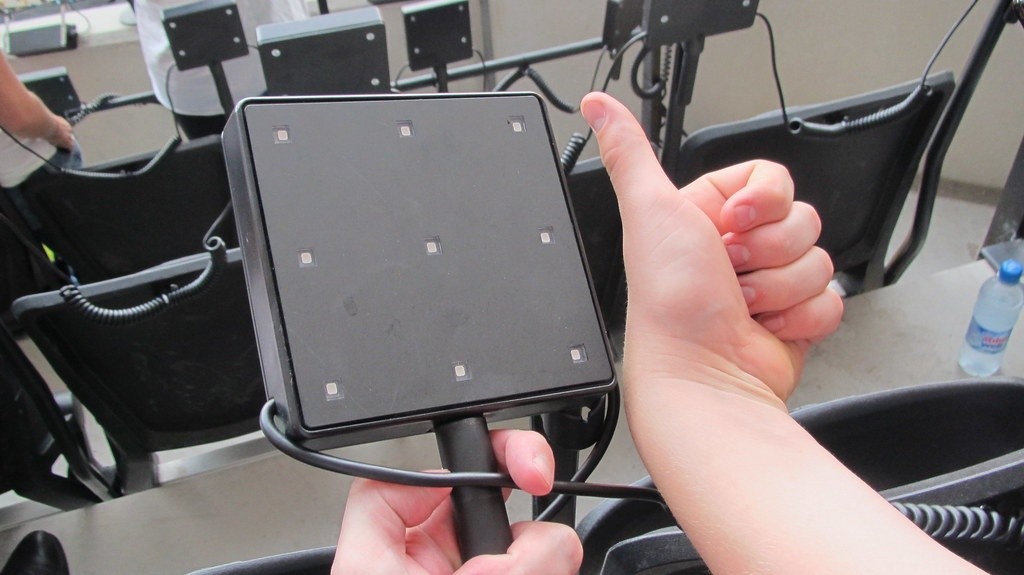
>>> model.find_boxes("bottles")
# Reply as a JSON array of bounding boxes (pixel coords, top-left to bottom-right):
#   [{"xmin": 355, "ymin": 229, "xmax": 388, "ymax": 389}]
[{"xmin": 957, "ymin": 259, "xmax": 1024, "ymax": 376}]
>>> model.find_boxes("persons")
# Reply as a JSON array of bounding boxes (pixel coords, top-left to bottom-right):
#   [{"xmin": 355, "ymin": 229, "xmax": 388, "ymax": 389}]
[
  {"xmin": 580, "ymin": 91, "xmax": 992, "ymax": 575},
  {"xmin": 331, "ymin": 426, "xmax": 584, "ymax": 575},
  {"xmin": 0, "ymin": 49, "xmax": 85, "ymax": 251},
  {"xmin": 134, "ymin": 0, "xmax": 314, "ymax": 143}
]
